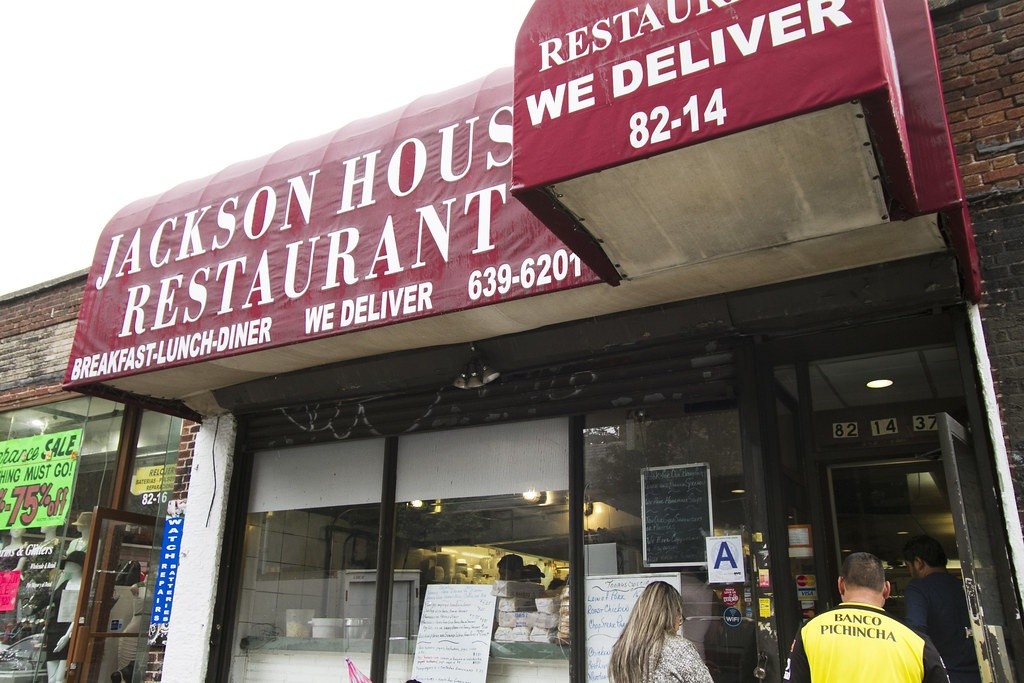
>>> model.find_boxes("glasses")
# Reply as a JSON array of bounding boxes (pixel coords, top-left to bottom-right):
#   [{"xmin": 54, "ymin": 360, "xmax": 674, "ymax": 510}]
[{"xmin": 678, "ymin": 610, "xmax": 686, "ymax": 626}]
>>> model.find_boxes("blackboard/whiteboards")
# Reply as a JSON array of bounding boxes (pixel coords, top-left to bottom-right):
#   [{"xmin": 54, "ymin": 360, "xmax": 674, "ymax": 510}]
[
  {"xmin": 640, "ymin": 462, "xmax": 714, "ymax": 568},
  {"xmin": 585, "ymin": 572, "xmax": 683, "ymax": 683}
]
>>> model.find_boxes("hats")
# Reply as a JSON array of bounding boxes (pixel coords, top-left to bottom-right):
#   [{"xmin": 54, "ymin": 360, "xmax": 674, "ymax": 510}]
[
  {"xmin": 523, "ymin": 565, "xmax": 545, "ymax": 579},
  {"xmin": 60, "ymin": 551, "xmax": 86, "ymax": 572},
  {"xmin": 72, "ymin": 512, "xmax": 93, "ymax": 527}
]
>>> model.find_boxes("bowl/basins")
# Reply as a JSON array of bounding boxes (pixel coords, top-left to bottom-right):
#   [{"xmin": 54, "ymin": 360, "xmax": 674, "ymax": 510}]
[
  {"xmin": 312, "ymin": 618, "xmax": 343, "ymax": 639},
  {"xmin": 343, "ymin": 618, "xmax": 369, "ymax": 639}
]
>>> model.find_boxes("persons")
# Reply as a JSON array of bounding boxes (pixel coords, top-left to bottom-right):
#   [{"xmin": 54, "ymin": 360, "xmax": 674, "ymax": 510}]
[
  {"xmin": 0, "ymin": 512, "xmax": 149, "ymax": 683},
  {"xmin": 608, "ymin": 580, "xmax": 713, "ymax": 683},
  {"xmin": 782, "ymin": 552, "xmax": 952, "ymax": 683},
  {"xmin": 902, "ymin": 535, "xmax": 983, "ymax": 683},
  {"xmin": 496, "ymin": 554, "xmax": 567, "ymax": 625}
]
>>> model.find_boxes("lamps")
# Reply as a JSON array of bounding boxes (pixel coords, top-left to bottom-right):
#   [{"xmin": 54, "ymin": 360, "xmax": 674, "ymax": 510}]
[{"xmin": 452, "ymin": 342, "xmax": 500, "ymax": 390}]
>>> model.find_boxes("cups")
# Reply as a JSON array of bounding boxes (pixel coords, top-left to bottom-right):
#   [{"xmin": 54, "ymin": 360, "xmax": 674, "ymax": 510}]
[{"xmin": 285, "ymin": 608, "xmax": 315, "ymax": 639}]
